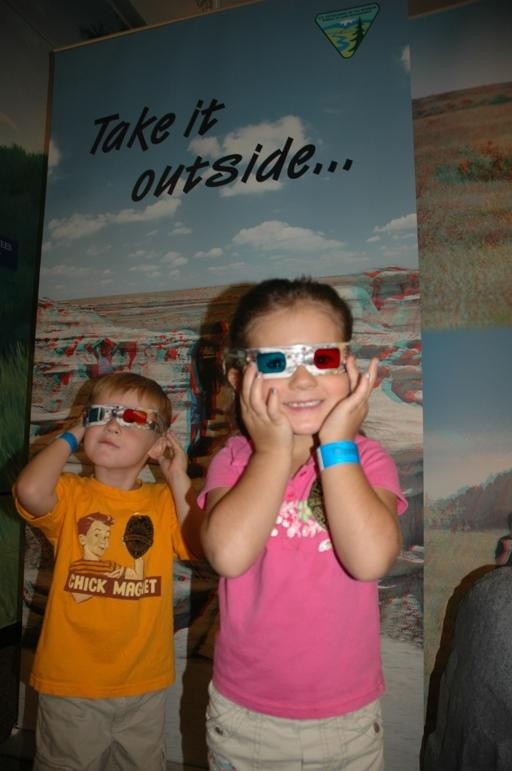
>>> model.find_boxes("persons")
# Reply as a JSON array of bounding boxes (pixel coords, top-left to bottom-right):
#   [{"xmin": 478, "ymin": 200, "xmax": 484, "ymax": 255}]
[
  {"xmin": 9, "ymin": 368, "xmax": 207, "ymax": 771},
  {"xmin": 67, "ymin": 509, "xmax": 146, "ymax": 587},
  {"xmin": 192, "ymin": 265, "xmax": 410, "ymax": 768}
]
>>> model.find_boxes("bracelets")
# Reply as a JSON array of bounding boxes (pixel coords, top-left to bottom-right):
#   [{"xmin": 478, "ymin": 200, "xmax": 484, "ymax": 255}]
[
  {"xmin": 314, "ymin": 438, "xmax": 365, "ymax": 472},
  {"xmin": 48, "ymin": 429, "xmax": 79, "ymax": 456}
]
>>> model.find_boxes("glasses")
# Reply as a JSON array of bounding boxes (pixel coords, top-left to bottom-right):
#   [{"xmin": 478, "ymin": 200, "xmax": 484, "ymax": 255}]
[
  {"xmin": 84, "ymin": 403, "xmax": 160, "ymax": 433},
  {"xmin": 246, "ymin": 339, "xmax": 353, "ymax": 382}
]
